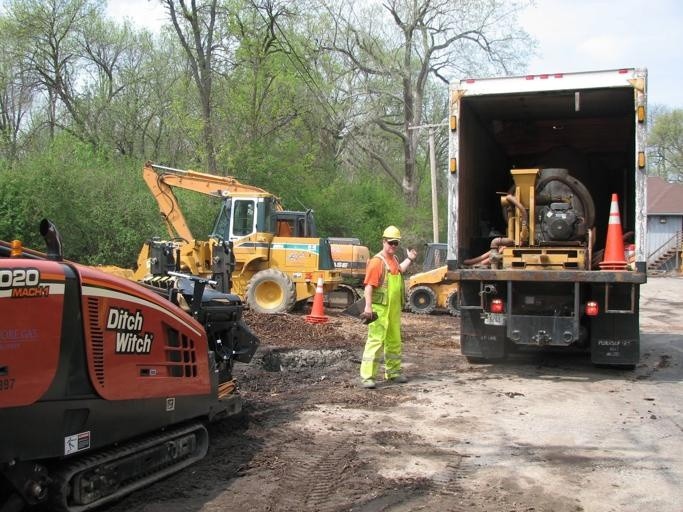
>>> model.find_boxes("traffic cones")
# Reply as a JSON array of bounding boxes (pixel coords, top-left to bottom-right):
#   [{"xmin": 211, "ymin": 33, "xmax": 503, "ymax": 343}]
[
  {"xmin": 600, "ymin": 184, "xmax": 635, "ymax": 271},
  {"xmin": 306, "ymin": 273, "xmax": 329, "ymax": 324}
]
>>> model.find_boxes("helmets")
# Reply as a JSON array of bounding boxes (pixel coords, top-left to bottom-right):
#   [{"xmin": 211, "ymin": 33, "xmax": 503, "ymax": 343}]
[{"xmin": 382, "ymin": 225, "xmax": 402, "ymax": 243}]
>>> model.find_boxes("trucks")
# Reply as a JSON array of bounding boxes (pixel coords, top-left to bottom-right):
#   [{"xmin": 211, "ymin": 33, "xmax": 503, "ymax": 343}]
[{"xmin": 446, "ymin": 65, "xmax": 649, "ymax": 374}]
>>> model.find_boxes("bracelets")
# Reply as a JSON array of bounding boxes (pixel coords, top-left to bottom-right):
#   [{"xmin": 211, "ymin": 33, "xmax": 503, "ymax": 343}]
[{"xmin": 406, "ymin": 256, "xmax": 413, "ymax": 263}]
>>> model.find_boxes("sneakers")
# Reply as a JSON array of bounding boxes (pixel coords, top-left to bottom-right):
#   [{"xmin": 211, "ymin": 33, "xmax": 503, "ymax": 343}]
[
  {"xmin": 390, "ymin": 374, "xmax": 408, "ymax": 382},
  {"xmin": 363, "ymin": 383, "xmax": 376, "ymax": 388}
]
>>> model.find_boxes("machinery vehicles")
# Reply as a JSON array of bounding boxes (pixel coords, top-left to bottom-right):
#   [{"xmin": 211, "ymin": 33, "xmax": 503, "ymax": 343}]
[
  {"xmin": 402, "ymin": 232, "xmax": 460, "ymax": 316},
  {"xmin": 0, "ymin": 216, "xmax": 261, "ymax": 512},
  {"xmin": 133, "ymin": 160, "xmax": 371, "ymax": 316}
]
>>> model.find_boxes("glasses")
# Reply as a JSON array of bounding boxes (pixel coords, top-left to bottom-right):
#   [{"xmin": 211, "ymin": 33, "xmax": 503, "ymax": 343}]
[{"xmin": 386, "ymin": 241, "xmax": 399, "ymax": 247}]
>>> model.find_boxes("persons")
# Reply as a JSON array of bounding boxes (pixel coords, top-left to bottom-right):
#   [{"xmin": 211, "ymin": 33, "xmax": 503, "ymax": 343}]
[{"xmin": 359, "ymin": 224, "xmax": 418, "ymax": 386}]
